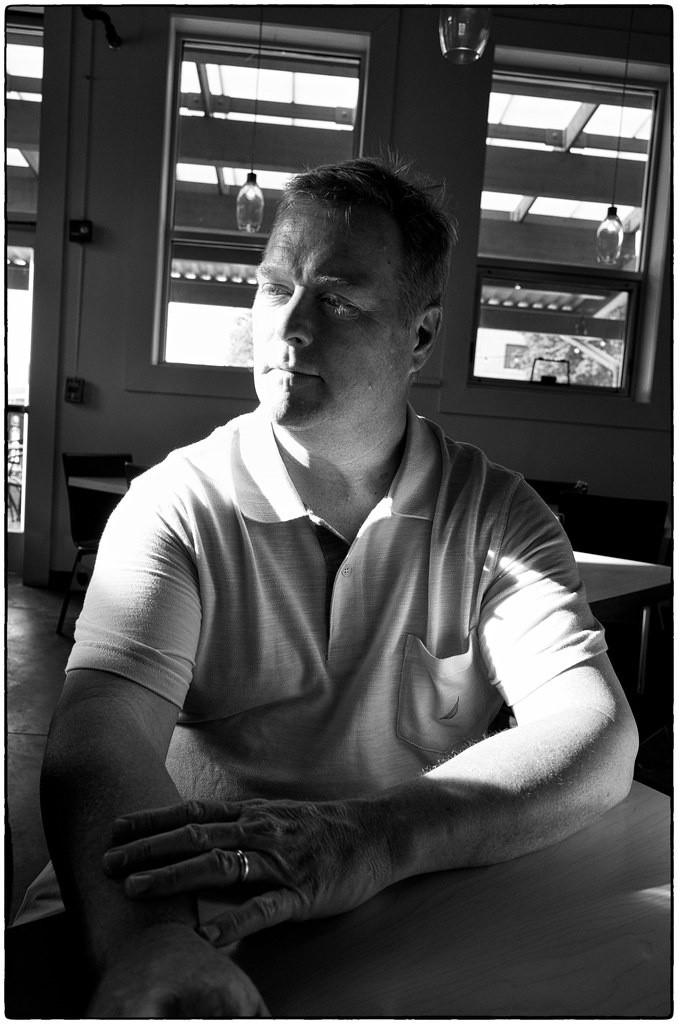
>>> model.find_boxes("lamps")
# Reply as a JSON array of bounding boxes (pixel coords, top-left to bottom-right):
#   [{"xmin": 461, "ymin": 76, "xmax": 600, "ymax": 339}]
[
  {"xmin": 596, "ymin": 62, "xmax": 629, "ymax": 265},
  {"xmin": 235, "ymin": 5, "xmax": 263, "ymax": 233}
]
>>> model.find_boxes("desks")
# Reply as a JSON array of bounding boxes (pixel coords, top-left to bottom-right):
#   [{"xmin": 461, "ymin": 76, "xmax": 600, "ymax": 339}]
[
  {"xmin": 221, "ymin": 779, "xmax": 672, "ymax": 1018},
  {"xmin": 572, "ymin": 550, "xmax": 671, "ymax": 604},
  {"xmin": 67, "ymin": 476, "xmax": 130, "ymax": 494}
]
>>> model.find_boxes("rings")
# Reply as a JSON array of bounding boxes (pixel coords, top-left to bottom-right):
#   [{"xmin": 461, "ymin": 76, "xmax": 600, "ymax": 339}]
[{"xmin": 235, "ymin": 847, "xmax": 249, "ymax": 884}]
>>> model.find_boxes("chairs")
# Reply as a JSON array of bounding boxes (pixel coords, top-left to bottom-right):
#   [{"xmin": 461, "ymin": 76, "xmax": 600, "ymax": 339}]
[
  {"xmin": 523, "ymin": 477, "xmax": 673, "ymax": 695},
  {"xmin": 55, "ymin": 451, "xmax": 133, "ymax": 635}
]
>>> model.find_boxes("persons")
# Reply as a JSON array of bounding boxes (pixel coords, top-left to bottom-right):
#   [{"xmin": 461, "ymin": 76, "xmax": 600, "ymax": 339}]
[{"xmin": 41, "ymin": 154, "xmax": 641, "ymax": 1020}]
